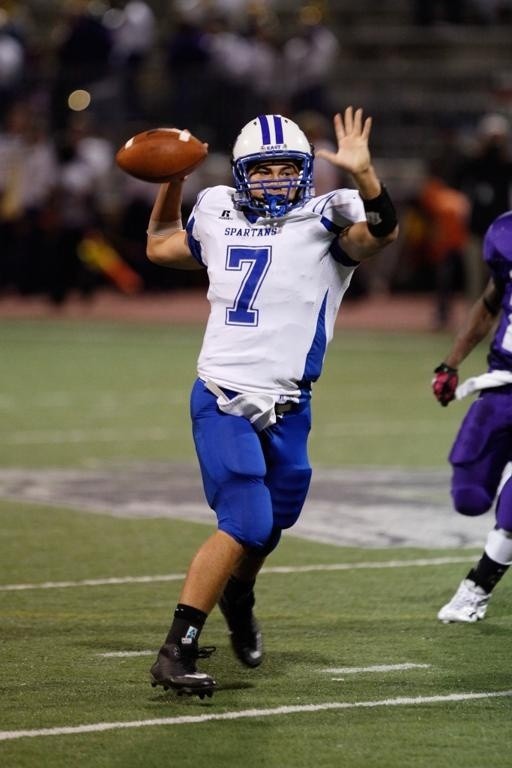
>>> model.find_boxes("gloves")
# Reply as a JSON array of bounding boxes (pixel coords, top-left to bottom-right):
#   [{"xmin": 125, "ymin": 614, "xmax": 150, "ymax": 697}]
[{"xmin": 428, "ymin": 360, "xmax": 463, "ymax": 409}]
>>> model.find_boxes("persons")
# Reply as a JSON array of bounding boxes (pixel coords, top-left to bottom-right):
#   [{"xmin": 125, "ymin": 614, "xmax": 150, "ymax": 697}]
[
  {"xmin": 144, "ymin": 104, "xmax": 399, "ymax": 700},
  {"xmin": 431, "ymin": 210, "xmax": 512, "ymax": 624}
]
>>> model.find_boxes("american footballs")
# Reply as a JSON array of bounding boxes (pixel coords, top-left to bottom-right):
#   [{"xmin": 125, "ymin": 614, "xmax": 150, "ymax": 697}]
[{"xmin": 116, "ymin": 126, "xmax": 208, "ymax": 183}]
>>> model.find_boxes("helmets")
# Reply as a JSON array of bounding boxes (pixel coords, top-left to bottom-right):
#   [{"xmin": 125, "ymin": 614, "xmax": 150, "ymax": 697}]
[{"xmin": 231, "ymin": 112, "xmax": 315, "ymax": 219}]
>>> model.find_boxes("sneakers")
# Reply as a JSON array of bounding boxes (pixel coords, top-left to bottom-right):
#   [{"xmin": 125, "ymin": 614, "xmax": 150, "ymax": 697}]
[
  {"xmin": 216, "ymin": 581, "xmax": 265, "ymax": 668},
  {"xmin": 148, "ymin": 642, "xmax": 218, "ymax": 700},
  {"xmin": 436, "ymin": 567, "xmax": 493, "ymax": 624}
]
